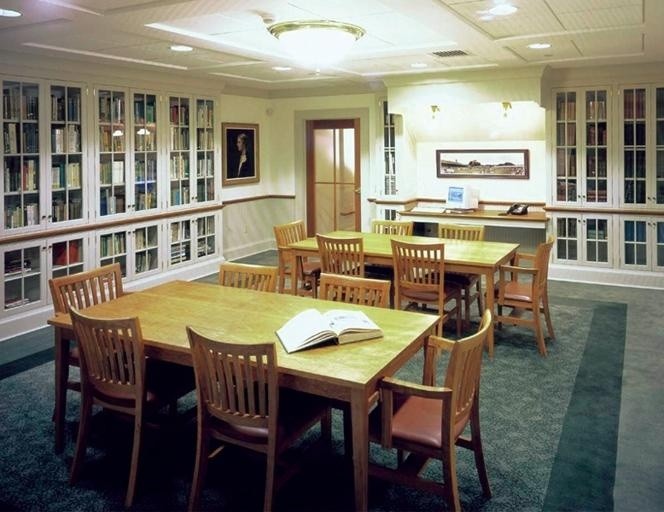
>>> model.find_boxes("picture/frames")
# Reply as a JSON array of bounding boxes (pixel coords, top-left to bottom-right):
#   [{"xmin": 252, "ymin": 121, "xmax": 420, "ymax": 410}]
[
  {"xmin": 221, "ymin": 122, "xmax": 260, "ymax": 188},
  {"xmin": 436, "ymin": 149, "xmax": 529, "ymax": 180}
]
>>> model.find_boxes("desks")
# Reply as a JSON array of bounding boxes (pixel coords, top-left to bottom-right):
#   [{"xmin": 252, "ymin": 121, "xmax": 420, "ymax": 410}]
[{"xmin": 400, "ymin": 211, "xmax": 548, "ymax": 229}]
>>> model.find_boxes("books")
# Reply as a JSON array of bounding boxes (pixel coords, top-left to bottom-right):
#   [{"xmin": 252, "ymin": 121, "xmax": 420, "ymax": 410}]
[
  {"xmin": 274, "ymin": 308, "xmax": 384, "ymax": 354},
  {"xmin": 2, "ymin": 78, "xmax": 83, "ymax": 309},
  {"xmin": 98, "ymin": 90, "xmax": 158, "ymax": 285},
  {"xmin": 556, "ymin": 92, "xmax": 646, "ymax": 243},
  {"xmin": 169, "ymin": 96, "xmax": 216, "ymax": 264}
]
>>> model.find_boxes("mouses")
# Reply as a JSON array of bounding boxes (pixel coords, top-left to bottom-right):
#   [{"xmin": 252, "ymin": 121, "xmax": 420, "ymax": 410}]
[{"xmin": 446, "ymin": 209, "xmax": 452, "ymax": 214}]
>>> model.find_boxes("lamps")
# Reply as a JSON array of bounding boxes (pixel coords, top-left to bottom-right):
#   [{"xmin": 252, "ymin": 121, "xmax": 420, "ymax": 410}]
[{"xmin": 267, "ymin": 21, "xmax": 365, "ymax": 77}]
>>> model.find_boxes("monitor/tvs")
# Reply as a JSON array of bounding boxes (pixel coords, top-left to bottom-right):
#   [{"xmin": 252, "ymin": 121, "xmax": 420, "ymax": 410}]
[{"xmin": 445, "ymin": 184, "xmax": 479, "ymax": 213}]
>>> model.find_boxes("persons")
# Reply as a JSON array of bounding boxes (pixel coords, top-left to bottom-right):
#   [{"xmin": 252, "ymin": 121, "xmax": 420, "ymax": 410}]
[{"xmin": 236, "ymin": 134, "xmax": 252, "ymax": 178}]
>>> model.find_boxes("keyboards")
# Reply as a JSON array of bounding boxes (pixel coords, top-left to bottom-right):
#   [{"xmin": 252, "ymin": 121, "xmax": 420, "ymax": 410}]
[{"xmin": 410, "ymin": 207, "xmax": 446, "ymax": 214}]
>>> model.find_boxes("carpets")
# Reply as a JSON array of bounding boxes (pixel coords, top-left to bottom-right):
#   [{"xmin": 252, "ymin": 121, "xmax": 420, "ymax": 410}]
[{"xmin": 0, "ymin": 270, "xmax": 627, "ymax": 511}]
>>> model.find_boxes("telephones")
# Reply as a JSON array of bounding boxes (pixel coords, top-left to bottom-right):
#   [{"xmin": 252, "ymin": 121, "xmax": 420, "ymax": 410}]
[{"xmin": 507, "ymin": 203, "xmax": 528, "ymax": 215}]
